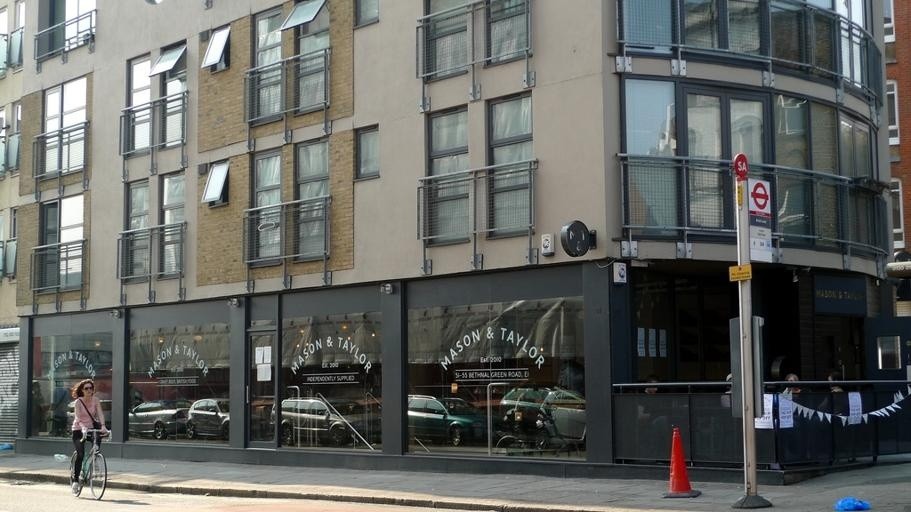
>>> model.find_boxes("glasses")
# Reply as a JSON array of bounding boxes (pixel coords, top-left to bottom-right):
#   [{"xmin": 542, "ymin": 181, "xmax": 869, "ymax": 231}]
[{"xmin": 83, "ymin": 387, "xmax": 92, "ymax": 389}]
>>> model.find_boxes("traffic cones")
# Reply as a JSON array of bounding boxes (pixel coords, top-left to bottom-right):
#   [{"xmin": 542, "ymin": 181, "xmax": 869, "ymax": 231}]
[{"xmin": 661, "ymin": 423, "xmax": 704, "ymax": 501}]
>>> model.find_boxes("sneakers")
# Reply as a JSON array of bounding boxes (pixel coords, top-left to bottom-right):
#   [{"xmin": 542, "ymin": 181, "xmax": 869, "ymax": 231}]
[{"xmin": 72, "ymin": 482, "xmax": 78, "ymax": 493}]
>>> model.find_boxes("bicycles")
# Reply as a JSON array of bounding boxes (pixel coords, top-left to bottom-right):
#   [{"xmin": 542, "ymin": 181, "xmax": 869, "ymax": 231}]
[
  {"xmin": 68, "ymin": 428, "xmax": 113, "ymax": 501},
  {"xmin": 493, "ymin": 396, "xmax": 580, "ymax": 458}
]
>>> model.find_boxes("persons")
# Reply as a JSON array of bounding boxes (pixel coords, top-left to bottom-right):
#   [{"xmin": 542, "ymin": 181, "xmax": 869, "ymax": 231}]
[
  {"xmin": 720, "ymin": 373, "xmax": 733, "ymax": 408},
  {"xmin": 777, "ymin": 372, "xmax": 814, "ymax": 394},
  {"xmin": 637, "ymin": 377, "xmax": 659, "ymax": 421},
  {"xmin": 816, "ymin": 371, "xmax": 850, "ymax": 411},
  {"xmin": 71, "ymin": 378, "xmax": 109, "ymax": 494}
]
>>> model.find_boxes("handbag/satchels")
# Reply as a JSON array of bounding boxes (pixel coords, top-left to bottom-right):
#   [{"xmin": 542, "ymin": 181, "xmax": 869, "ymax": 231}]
[{"xmin": 93, "ymin": 421, "xmax": 111, "ymax": 435}]
